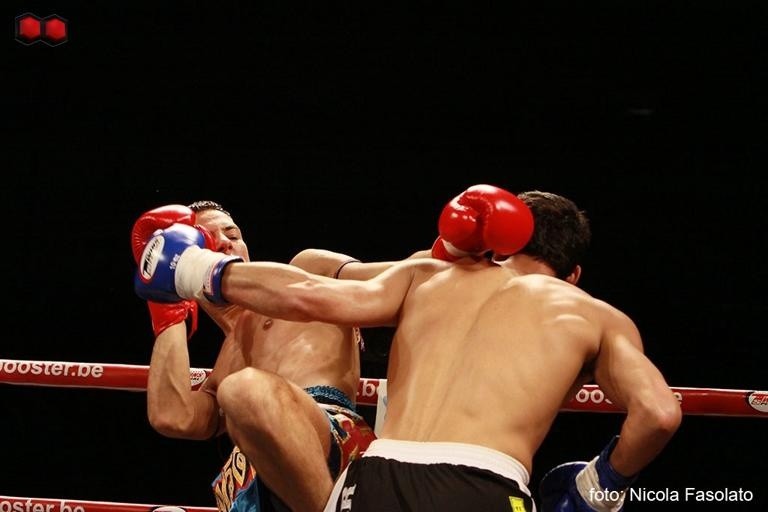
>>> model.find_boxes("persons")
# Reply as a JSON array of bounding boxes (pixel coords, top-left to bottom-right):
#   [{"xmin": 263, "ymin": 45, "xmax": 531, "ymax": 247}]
[
  {"xmin": 133, "ymin": 190, "xmax": 684, "ymax": 510},
  {"xmin": 131, "ymin": 177, "xmax": 537, "ymax": 511}
]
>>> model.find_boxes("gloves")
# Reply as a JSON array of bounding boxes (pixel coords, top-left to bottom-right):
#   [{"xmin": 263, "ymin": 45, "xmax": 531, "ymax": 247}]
[
  {"xmin": 135, "ymin": 224, "xmax": 245, "ymax": 306},
  {"xmin": 429, "ymin": 183, "xmax": 536, "ymax": 264},
  {"xmin": 537, "ymin": 433, "xmax": 630, "ymax": 512},
  {"xmin": 128, "ymin": 203, "xmax": 217, "ymax": 344}
]
